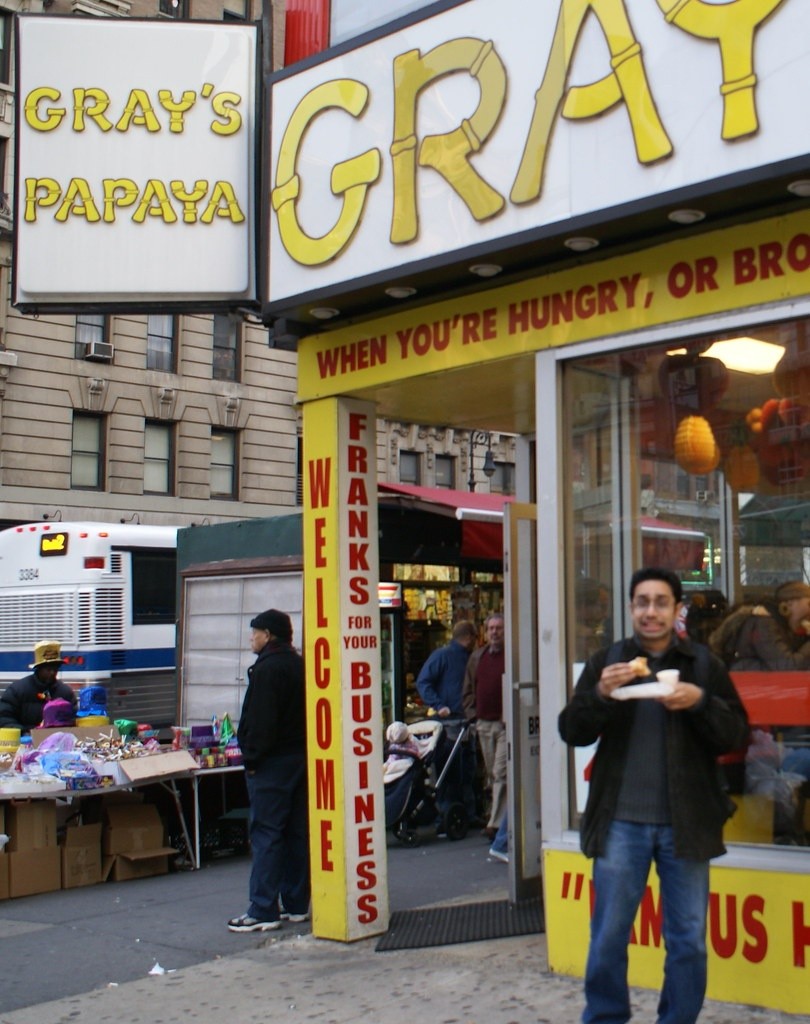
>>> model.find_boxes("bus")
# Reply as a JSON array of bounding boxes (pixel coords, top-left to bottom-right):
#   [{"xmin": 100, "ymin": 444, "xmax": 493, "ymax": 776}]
[{"xmin": 0, "ymin": 522, "xmax": 182, "ymax": 742}]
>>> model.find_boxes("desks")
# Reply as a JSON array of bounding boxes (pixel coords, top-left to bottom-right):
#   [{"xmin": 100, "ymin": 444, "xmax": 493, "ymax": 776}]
[
  {"xmin": 0, "ymin": 771, "xmax": 197, "ymax": 869},
  {"xmin": 181, "ymin": 764, "xmax": 245, "ymax": 868}
]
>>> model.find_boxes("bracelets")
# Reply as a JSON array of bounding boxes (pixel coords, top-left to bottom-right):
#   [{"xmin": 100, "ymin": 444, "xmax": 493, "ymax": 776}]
[{"xmin": 693, "ymin": 682, "xmax": 705, "ymax": 706}]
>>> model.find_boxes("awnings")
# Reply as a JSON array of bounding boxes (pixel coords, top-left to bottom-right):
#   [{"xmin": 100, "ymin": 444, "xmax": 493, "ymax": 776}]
[{"xmin": 377, "ymin": 483, "xmax": 706, "ymax": 570}]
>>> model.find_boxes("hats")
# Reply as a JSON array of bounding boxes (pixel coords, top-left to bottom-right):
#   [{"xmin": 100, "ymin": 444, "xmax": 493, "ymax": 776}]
[
  {"xmin": 36, "ymin": 700, "xmax": 76, "ymax": 728},
  {"xmin": 27, "ymin": 642, "xmax": 68, "ymax": 668},
  {"xmin": 386, "ymin": 721, "xmax": 408, "ymax": 743},
  {"xmin": 75, "ymin": 686, "xmax": 108, "ymax": 716},
  {"xmin": 250, "ymin": 609, "xmax": 293, "ymax": 641}
]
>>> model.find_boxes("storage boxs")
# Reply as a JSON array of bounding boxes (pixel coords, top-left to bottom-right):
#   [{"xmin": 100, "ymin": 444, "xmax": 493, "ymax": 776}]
[{"xmin": 0, "ymin": 725, "xmax": 201, "ymax": 901}]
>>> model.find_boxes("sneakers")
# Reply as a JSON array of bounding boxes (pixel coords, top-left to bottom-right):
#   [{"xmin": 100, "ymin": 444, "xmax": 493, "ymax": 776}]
[
  {"xmin": 228, "ymin": 912, "xmax": 281, "ymax": 932},
  {"xmin": 279, "ymin": 907, "xmax": 307, "ymax": 922}
]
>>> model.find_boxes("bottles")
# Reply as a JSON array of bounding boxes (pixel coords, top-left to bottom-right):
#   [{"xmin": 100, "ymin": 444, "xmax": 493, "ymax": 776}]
[
  {"xmin": 173, "ymin": 727, "xmax": 228, "ymax": 769},
  {"xmin": 20, "ymin": 736, "xmax": 34, "ymax": 774}
]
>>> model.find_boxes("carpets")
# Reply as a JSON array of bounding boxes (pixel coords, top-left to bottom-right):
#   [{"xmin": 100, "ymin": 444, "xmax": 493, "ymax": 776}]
[{"xmin": 375, "ymin": 895, "xmax": 546, "ymax": 952}]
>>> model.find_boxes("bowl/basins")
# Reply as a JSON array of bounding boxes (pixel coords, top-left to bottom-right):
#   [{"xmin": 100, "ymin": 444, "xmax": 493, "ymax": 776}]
[{"xmin": 610, "ymin": 681, "xmax": 676, "ymax": 700}]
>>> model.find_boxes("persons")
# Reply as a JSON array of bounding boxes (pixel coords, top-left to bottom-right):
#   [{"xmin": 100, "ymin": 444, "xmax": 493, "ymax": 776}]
[
  {"xmin": 414, "ymin": 620, "xmax": 484, "ymax": 840},
  {"xmin": 489, "ymin": 578, "xmax": 609, "ymax": 864},
  {"xmin": 227, "ymin": 608, "xmax": 312, "ymax": 932},
  {"xmin": 460, "ymin": 614, "xmax": 505, "ymax": 842},
  {"xmin": 673, "ymin": 580, "xmax": 810, "ymax": 671},
  {"xmin": 0, "ymin": 641, "xmax": 78, "ymax": 737},
  {"xmin": 558, "ymin": 567, "xmax": 754, "ymax": 1024}
]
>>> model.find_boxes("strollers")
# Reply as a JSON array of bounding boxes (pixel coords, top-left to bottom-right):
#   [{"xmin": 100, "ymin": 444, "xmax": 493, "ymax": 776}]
[{"xmin": 383, "ymin": 711, "xmax": 481, "ymax": 848}]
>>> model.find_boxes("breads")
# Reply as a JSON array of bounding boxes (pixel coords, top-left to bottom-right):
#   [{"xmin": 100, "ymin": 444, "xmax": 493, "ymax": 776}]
[{"xmin": 628, "ymin": 656, "xmax": 651, "ymax": 676}]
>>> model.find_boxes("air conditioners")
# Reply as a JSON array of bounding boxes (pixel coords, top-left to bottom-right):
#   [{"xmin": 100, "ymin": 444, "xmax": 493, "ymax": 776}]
[{"xmin": 86, "ymin": 342, "xmax": 114, "ymax": 358}]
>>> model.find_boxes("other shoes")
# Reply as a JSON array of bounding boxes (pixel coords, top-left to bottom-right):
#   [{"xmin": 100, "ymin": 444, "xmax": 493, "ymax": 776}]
[{"xmin": 490, "ymin": 847, "xmax": 509, "ymax": 863}]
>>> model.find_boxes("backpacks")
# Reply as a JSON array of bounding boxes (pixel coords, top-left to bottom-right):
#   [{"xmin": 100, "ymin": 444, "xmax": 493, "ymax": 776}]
[{"xmin": 709, "ymin": 604, "xmax": 758, "ymax": 662}]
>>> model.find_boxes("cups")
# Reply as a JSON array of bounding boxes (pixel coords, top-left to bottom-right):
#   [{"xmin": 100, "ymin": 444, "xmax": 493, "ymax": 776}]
[{"xmin": 656, "ymin": 669, "xmax": 680, "ymax": 682}]
